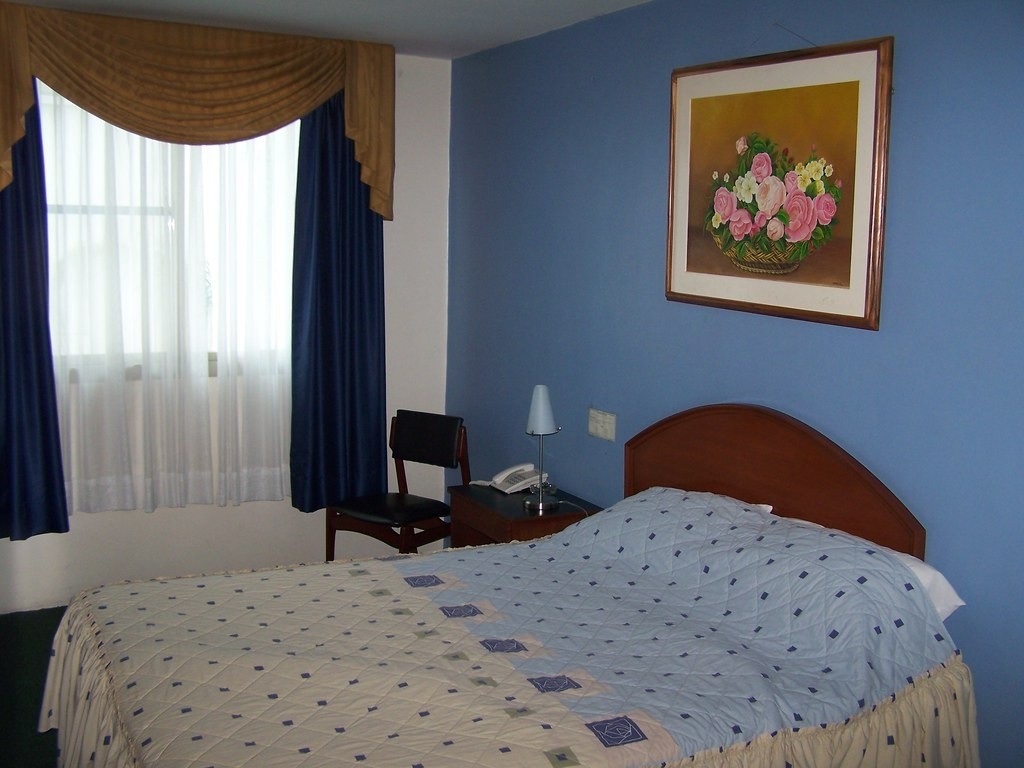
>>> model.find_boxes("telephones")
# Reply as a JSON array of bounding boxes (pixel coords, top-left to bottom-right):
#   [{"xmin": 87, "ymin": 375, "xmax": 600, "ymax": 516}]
[{"xmin": 490, "ymin": 462, "xmax": 549, "ymax": 495}]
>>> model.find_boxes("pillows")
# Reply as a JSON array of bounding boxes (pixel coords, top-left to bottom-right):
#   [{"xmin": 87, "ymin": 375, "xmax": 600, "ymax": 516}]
[{"xmin": 589, "ymin": 485, "xmax": 957, "ymax": 645}]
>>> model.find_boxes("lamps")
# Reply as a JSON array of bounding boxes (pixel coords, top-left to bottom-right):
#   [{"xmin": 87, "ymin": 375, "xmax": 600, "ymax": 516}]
[{"xmin": 516, "ymin": 386, "xmax": 558, "ymax": 497}]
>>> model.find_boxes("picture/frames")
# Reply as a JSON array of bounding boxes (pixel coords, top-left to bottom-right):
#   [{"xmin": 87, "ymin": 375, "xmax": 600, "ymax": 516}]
[{"xmin": 664, "ymin": 36, "xmax": 894, "ymax": 332}]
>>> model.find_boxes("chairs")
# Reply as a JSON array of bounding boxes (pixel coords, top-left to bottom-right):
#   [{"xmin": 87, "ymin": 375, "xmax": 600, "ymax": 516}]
[{"xmin": 325, "ymin": 408, "xmax": 470, "ymax": 562}]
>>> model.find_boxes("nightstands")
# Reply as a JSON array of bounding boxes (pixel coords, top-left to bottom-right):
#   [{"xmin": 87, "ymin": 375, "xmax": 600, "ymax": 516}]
[{"xmin": 446, "ymin": 483, "xmax": 601, "ymax": 549}]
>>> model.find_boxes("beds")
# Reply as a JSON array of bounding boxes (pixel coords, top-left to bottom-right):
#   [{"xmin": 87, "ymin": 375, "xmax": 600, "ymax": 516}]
[{"xmin": 40, "ymin": 403, "xmax": 980, "ymax": 768}]
